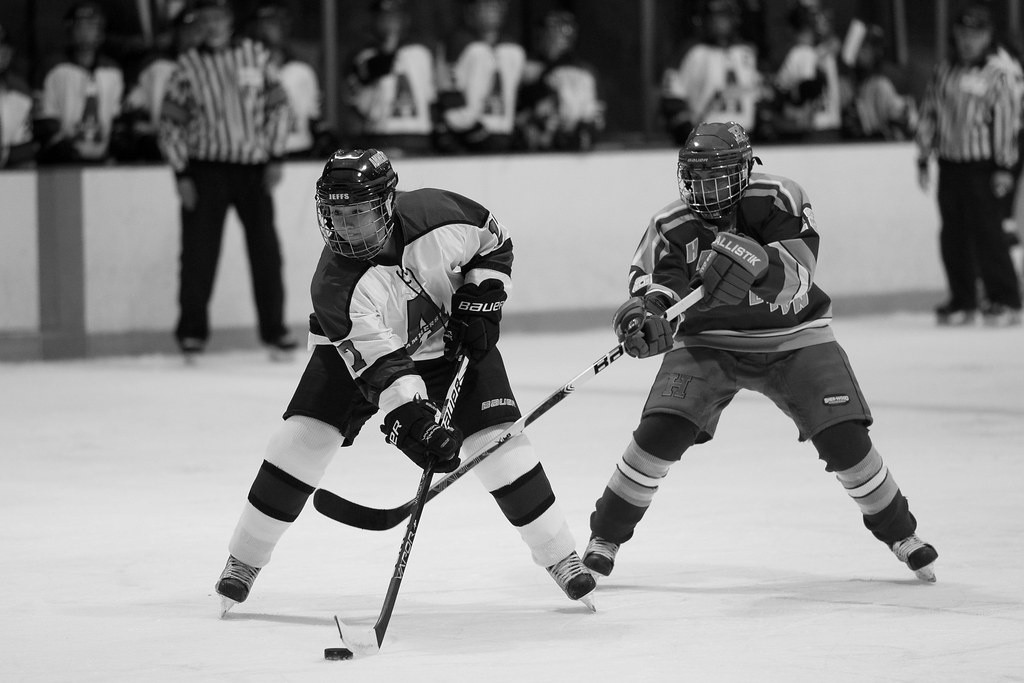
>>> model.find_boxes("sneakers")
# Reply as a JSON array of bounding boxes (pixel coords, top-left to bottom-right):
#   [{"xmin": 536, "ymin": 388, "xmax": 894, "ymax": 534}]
[
  {"xmin": 888, "ymin": 533, "xmax": 938, "ymax": 572},
  {"xmin": 581, "ymin": 532, "xmax": 619, "ymax": 576},
  {"xmin": 215, "ymin": 556, "xmax": 261, "ymax": 603},
  {"xmin": 934, "ymin": 295, "xmax": 979, "ymax": 316},
  {"xmin": 983, "ymin": 298, "xmax": 1022, "ymax": 316},
  {"xmin": 263, "ymin": 334, "xmax": 299, "ymax": 352},
  {"xmin": 545, "ymin": 550, "xmax": 596, "ymax": 601},
  {"xmin": 177, "ymin": 337, "xmax": 206, "ymax": 354}
]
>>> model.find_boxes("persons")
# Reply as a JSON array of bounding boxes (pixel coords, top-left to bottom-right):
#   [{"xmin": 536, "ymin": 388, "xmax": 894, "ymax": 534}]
[
  {"xmin": 914, "ymin": 8, "xmax": 1024, "ymax": 326},
  {"xmin": 158, "ymin": 0, "xmax": 300, "ymax": 362},
  {"xmin": 256, "ymin": 0, "xmax": 605, "ymax": 161},
  {"xmin": 582, "ymin": 121, "xmax": 941, "ymax": 586},
  {"xmin": 214, "ymin": 150, "xmax": 597, "ymax": 619},
  {"xmin": 0, "ymin": 0, "xmax": 179, "ymax": 166},
  {"xmin": 659, "ymin": 0, "xmax": 919, "ymax": 140}
]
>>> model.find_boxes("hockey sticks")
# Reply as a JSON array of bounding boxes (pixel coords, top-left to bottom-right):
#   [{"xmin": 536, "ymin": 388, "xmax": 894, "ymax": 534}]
[
  {"xmin": 322, "ymin": 358, "xmax": 470, "ymax": 659},
  {"xmin": 314, "ymin": 284, "xmax": 710, "ymax": 529}
]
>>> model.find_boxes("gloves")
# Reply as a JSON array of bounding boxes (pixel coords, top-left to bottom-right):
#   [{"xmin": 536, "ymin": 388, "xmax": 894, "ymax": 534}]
[
  {"xmin": 448, "ymin": 277, "xmax": 508, "ymax": 359},
  {"xmin": 612, "ymin": 283, "xmax": 685, "ymax": 359},
  {"xmin": 380, "ymin": 399, "xmax": 463, "ymax": 473},
  {"xmin": 690, "ymin": 231, "xmax": 770, "ymax": 311}
]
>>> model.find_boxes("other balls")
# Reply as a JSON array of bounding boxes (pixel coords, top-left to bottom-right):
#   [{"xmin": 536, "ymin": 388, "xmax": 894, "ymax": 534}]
[{"xmin": 322, "ymin": 645, "xmax": 353, "ymax": 661}]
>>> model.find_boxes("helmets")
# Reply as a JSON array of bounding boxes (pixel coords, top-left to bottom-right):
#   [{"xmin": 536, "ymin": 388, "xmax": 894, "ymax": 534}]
[
  {"xmin": 677, "ymin": 122, "xmax": 763, "ymax": 219},
  {"xmin": 315, "ymin": 148, "xmax": 398, "ymax": 260}
]
